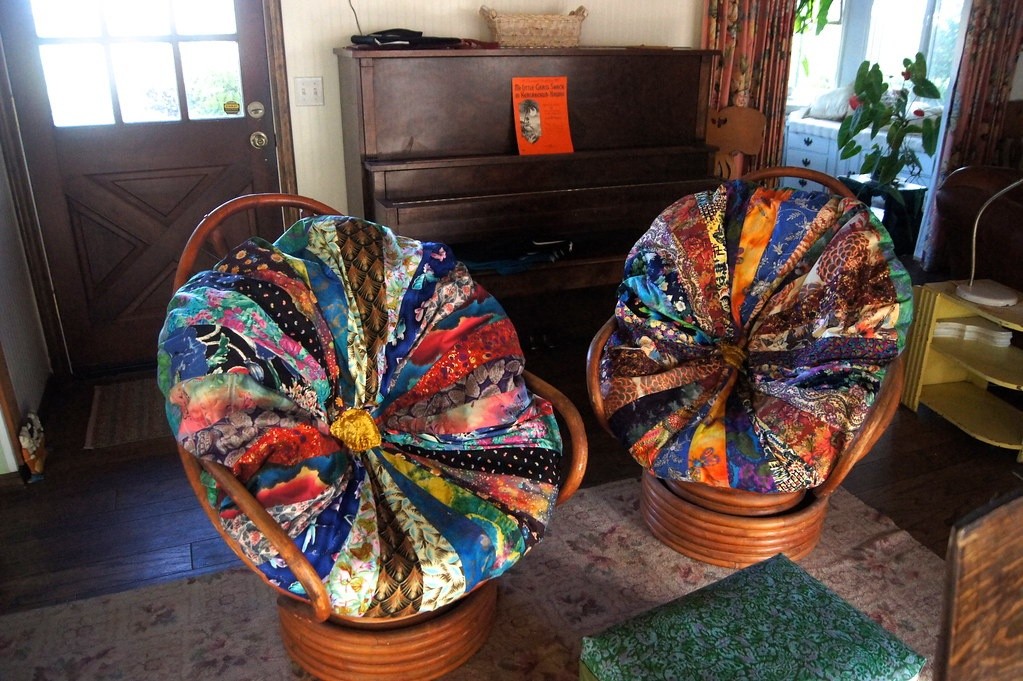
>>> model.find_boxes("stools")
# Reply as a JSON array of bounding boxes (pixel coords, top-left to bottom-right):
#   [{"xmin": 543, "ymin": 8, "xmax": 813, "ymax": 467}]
[{"xmin": 579, "ymin": 555, "xmax": 926, "ymax": 681}]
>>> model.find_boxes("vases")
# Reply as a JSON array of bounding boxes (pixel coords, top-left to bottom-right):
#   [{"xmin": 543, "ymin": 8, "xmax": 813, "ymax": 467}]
[{"xmin": 843, "ymin": 171, "xmax": 928, "ymax": 265}]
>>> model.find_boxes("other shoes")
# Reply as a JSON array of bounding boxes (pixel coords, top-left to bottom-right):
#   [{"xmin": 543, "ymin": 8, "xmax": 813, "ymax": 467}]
[
  {"xmin": 526, "ymin": 335, "xmax": 538, "ymax": 353},
  {"xmin": 539, "ymin": 334, "xmax": 560, "ymax": 351}
]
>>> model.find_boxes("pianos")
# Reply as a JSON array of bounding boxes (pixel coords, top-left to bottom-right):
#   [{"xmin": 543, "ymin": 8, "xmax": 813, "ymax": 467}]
[{"xmin": 331, "ymin": 44, "xmax": 731, "ymax": 368}]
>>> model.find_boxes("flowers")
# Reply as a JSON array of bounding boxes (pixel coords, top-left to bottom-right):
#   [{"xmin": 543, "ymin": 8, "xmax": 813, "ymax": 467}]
[{"xmin": 836, "ymin": 52, "xmax": 941, "ymax": 182}]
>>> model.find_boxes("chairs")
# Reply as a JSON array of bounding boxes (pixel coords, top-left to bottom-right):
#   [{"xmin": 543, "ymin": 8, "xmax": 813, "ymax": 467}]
[
  {"xmin": 588, "ymin": 167, "xmax": 906, "ymax": 569},
  {"xmin": 171, "ymin": 192, "xmax": 588, "ymax": 681}
]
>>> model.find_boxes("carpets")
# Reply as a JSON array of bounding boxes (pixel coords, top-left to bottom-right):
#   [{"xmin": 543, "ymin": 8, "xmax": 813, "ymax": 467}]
[
  {"xmin": 84, "ymin": 378, "xmax": 173, "ymax": 451},
  {"xmin": 0, "ymin": 467, "xmax": 949, "ymax": 681}
]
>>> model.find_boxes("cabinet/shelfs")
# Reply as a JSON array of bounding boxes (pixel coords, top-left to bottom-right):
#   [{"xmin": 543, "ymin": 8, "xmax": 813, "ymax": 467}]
[
  {"xmin": 783, "ymin": 123, "xmax": 940, "ymax": 259},
  {"xmin": 900, "ymin": 279, "xmax": 1023, "ymax": 461}
]
